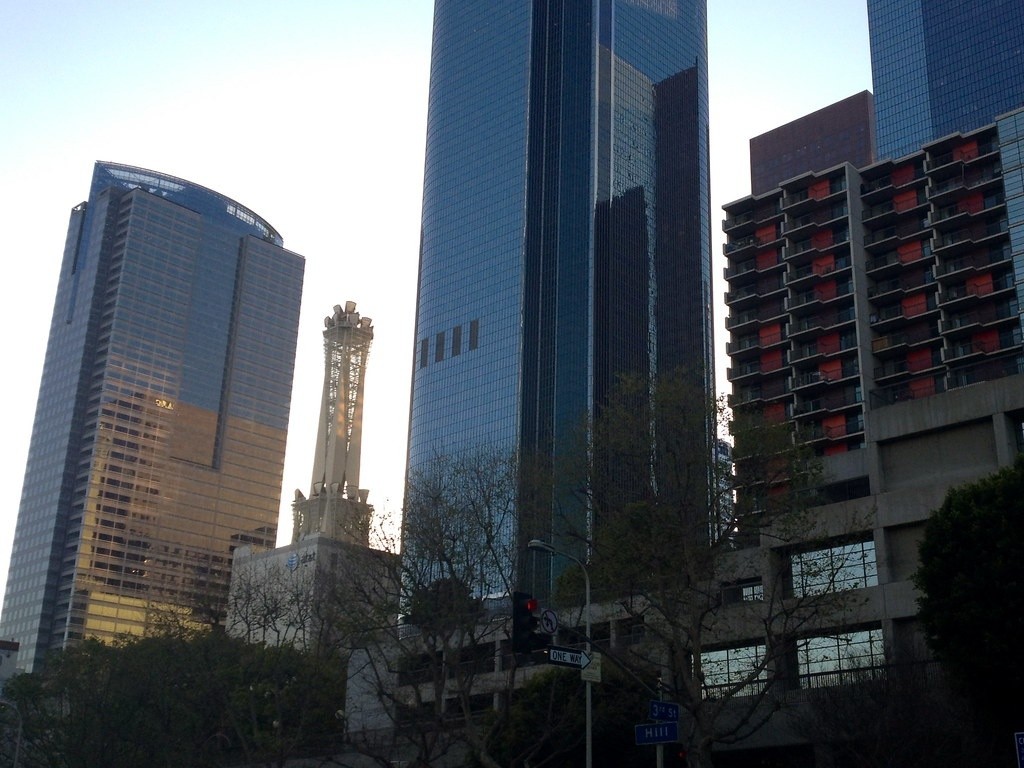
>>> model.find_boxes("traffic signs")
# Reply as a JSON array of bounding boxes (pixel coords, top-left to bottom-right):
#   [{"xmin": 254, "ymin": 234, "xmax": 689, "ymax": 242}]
[{"xmin": 543, "ymin": 643, "xmax": 592, "ymax": 670}]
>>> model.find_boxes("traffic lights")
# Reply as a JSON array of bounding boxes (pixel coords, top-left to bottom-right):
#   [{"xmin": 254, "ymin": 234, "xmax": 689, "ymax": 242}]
[{"xmin": 512, "ymin": 591, "xmax": 540, "ymax": 649}]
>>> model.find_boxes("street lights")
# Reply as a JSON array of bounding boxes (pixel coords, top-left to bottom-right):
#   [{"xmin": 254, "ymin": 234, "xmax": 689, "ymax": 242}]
[{"xmin": 528, "ymin": 540, "xmax": 593, "ymax": 768}]
